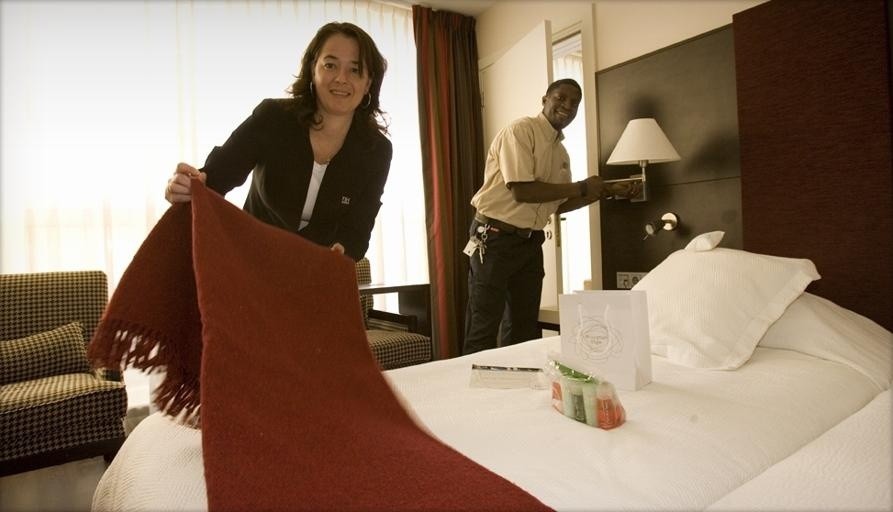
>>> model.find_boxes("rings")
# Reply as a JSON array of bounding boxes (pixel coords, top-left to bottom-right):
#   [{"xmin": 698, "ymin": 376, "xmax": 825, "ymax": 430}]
[{"xmin": 630, "ymin": 190, "xmax": 635, "ymax": 195}]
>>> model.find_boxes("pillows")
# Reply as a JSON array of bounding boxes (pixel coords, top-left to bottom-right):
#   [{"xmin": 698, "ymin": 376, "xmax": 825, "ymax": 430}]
[
  {"xmin": 623, "ymin": 247, "xmax": 822, "ymax": 371},
  {"xmin": 682, "ymin": 231, "xmax": 726, "ymax": 250},
  {"xmin": 0, "ymin": 321, "xmax": 93, "ymax": 386}
]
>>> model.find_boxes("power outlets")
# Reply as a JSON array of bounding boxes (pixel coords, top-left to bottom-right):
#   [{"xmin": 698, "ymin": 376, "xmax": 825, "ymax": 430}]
[{"xmin": 616, "ymin": 272, "xmax": 647, "ymax": 289}]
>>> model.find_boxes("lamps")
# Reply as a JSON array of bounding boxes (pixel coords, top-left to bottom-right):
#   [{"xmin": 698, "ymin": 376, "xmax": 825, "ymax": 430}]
[
  {"xmin": 607, "ymin": 114, "xmax": 680, "ymax": 203},
  {"xmin": 645, "ymin": 212, "xmax": 679, "ymax": 235}
]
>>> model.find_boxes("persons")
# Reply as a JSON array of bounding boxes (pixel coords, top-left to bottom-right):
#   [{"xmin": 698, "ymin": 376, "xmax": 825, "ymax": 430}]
[
  {"xmin": 460, "ymin": 77, "xmax": 645, "ymax": 356},
  {"xmin": 164, "ymin": 22, "xmax": 393, "ymax": 263}
]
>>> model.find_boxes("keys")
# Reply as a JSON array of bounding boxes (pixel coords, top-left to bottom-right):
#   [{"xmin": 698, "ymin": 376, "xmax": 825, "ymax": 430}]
[{"xmin": 472, "ymin": 223, "xmax": 490, "ymax": 264}]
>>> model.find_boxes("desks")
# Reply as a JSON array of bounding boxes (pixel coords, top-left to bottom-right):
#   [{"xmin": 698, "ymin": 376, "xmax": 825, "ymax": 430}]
[
  {"xmin": 536, "ymin": 309, "xmax": 559, "ymax": 339},
  {"xmin": 358, "ymin": 283, "xmax": 429, "ymax": 338}
]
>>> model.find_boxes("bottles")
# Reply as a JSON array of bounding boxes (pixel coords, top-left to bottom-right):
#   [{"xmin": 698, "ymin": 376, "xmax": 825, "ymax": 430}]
[{"xmin": 549, "ymin": 374, "xmax": 618, "ymax": 429}]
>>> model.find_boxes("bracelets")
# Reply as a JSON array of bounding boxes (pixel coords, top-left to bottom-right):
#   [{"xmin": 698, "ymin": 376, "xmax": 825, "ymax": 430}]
[{"xmin": 578, "ymin": 181, "xmax": 587, "ymax": 197}]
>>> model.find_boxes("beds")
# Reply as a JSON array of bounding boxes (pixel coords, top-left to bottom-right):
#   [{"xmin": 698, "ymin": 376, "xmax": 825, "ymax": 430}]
[{"xmin": 84, "ymin": 289, "xmax": 893, "ymax": 512}]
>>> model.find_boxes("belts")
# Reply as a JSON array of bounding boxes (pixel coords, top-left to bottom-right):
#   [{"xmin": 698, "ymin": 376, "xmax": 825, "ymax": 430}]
[{"xmin": 473, "ymin": 209, "xmax": 546, "ymax": 243}]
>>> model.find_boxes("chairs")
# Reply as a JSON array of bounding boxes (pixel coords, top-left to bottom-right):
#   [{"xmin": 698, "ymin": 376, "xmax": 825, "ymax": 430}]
[
  {"xmin": 352, "ymin": 257, "xmax": 433, "ymax": 373},
  {"xmin": 0, "ymin": 272, "xmax": 128, "ymax": 476}
]
